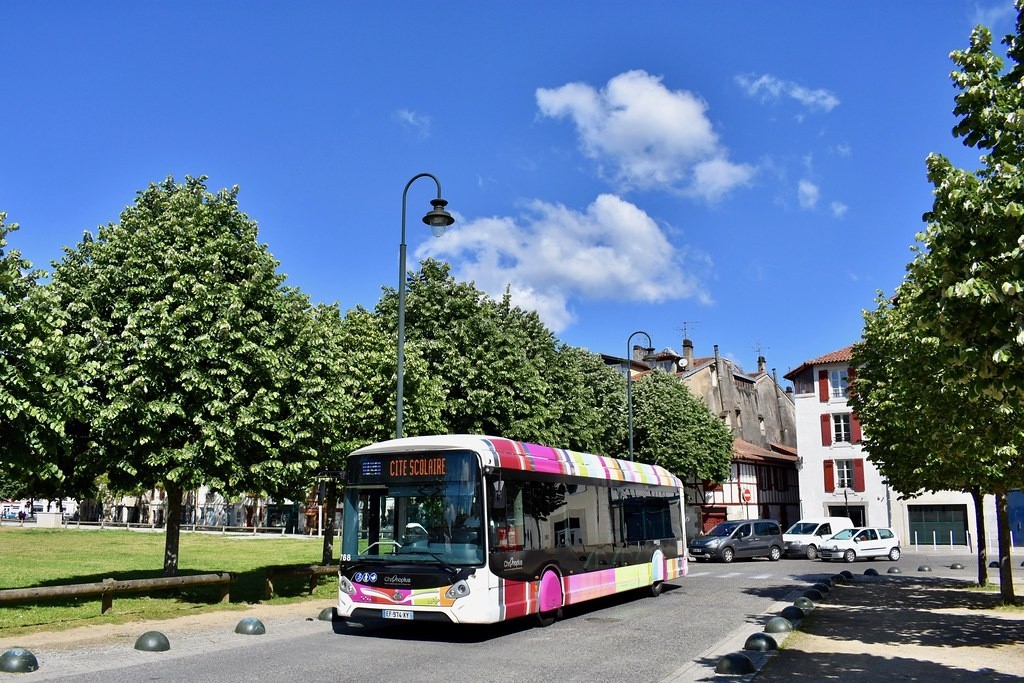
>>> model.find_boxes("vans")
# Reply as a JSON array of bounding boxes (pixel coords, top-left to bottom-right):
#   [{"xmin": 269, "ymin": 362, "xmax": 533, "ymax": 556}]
[{"xmin": 779, "ymin": 516, "xmax": 854, "ymax": 560}]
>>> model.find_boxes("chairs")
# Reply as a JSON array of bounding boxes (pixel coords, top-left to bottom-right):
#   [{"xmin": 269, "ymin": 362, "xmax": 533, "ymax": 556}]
[
  {"xmin": 819, "ymin": 526, "xmax": 828, "ymax": 534},
  {"xmin": 866, "ymin": 532, "xmax": 871, "ymax": 540},
  {"xmin": 450, "ymin": 514, "xmax": 483, "ymax": 547}
]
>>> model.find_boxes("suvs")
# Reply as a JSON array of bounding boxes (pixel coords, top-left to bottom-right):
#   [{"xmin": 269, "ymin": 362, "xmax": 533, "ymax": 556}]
[{"xmin": 688, "ymin": 519, "xmax": 786, "ymax": 564}]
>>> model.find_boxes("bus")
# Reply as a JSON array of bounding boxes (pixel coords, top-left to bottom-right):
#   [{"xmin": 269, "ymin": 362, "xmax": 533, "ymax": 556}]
[{"xmin": 314, "ymin": 433, "xmax": 689, "ymax": 628}]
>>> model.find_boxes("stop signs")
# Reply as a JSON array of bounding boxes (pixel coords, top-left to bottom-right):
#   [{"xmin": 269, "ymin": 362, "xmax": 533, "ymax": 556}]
[{"xmin": 743, "ymin": 488, "xmax": 752, "ymax": 503}]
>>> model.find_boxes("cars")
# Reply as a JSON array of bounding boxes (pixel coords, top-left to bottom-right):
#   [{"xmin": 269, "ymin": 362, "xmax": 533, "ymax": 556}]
[
  {"xmin": 0, "ymin": 511, "xmax": 39, "ymax": 520},
  {"xmin": 817, "ymin": 526, "xmax": 902, "ymax": 563},
  {"xmin": 62, "ymin": 512, "xmax": 75, "ymax": 524}
]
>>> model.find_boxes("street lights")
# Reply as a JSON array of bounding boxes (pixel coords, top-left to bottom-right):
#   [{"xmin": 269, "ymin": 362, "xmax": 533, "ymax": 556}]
[
  {"xmin": 395, "ymin": 171, "xmax": 456, "ymax": 440},
  {"xmin": 628, "ymin": 330, "xmax": 660, "ymax": 463}
]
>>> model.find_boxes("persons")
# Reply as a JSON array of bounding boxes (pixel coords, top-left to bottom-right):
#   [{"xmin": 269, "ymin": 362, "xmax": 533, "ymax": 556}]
[
  {"xmin": 462, "ymin": 504, "xmax": 495, "ymax": 538},
  {"xmin": 19, "ymin": 511, "xmax": 26, "ymax": 520},
  {"xmin": 860, "ymin": 533, "xmax": 866, "ymax": 540}
]
